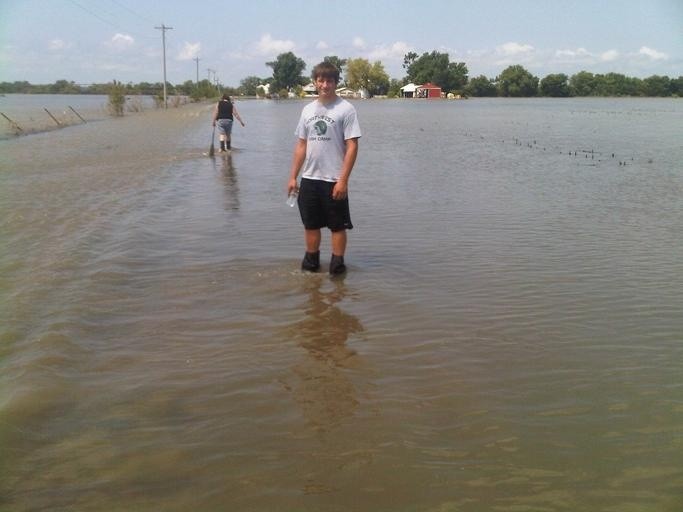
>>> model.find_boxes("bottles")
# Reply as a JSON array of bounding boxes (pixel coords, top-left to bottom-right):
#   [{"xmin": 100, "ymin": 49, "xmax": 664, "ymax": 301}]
[{"xmin": 285, "ymin": 182, "xmax": 300, "ymax": 208}]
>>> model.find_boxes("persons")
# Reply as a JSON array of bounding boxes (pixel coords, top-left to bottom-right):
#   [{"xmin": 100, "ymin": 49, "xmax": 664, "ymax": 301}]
[
  {"xmin": 286, "ymin": 60, "xmax": 362, "ymax": 274},
  {"xmin": 212, "ymin": 94, "xmax": 244, "ymax": 151}
]
